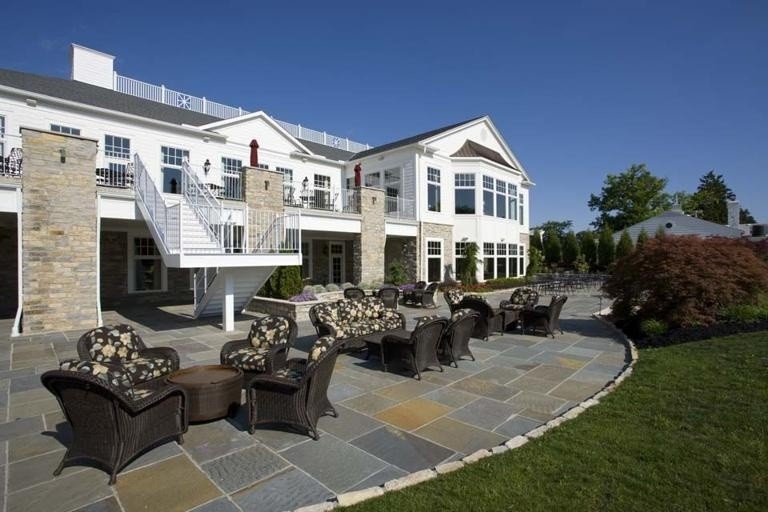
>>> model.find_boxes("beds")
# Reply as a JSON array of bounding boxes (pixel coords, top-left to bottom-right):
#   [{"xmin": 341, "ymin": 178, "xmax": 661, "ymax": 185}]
[{"xmin": 219, "ymin": 314, "xmax": 346, "ymax": 441}]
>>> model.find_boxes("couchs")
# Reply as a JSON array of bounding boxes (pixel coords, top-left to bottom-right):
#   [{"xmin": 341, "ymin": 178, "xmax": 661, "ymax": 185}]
[{"xmin": 308, "ymin": 295, "xmax": 408, "ymax": 353}]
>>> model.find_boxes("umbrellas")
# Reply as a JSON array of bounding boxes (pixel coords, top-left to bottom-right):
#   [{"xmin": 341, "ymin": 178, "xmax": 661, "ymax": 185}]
[
  {"xmin": 354, "ymin": 162, "xmax": 361, "ymax": 187},
  {"xmin": 250, "ymin": 140, "xmax": 258, "ymax": 167}
]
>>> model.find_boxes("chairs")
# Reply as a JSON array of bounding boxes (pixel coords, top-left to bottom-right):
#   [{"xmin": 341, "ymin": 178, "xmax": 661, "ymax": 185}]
[
  {"xmin": 400, "ymin": 280, "xmax": 438, "ymax": 310},
  {"xmin": 344, "ymin": 287, "xmax": 399, "ymax": 309},
  {"xmin": 37, "ymin": 323, "xmax": 190, "ymax": 487},
  {"xmin": 379, "ymin": 309, "xmax": 479, "ymax": 380},
  {"xmin": 443, "ymin": 287, "xmax": 568, "ymax": 342}
]
